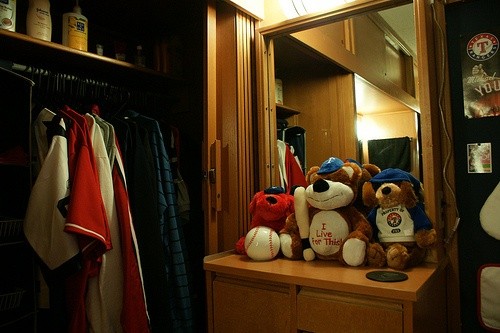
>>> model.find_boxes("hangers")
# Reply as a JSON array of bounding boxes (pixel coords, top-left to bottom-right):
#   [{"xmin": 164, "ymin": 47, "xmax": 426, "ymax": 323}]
[{"xmin": 25, "ymin": 67, "xmax": 176, "ymax": 126}]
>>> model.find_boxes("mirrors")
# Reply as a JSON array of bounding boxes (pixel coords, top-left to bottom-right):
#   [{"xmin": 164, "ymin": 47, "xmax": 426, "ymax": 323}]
[{"xmin": 256, "ymin": 0, "xmax": 428, "ymax": 220}]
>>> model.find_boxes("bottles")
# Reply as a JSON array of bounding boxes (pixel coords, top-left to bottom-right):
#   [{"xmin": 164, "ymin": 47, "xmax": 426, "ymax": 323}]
[
  {"xmin": 135, "ymin": 46, "xmax": 146, "ymax": 68},
  {"xmin": 0, "ymin": 0, "xmax": 16, "ymax": 32},
  {"xmin": 61, "ymin": 1, "xmax": 88, "ymax": 52},
  {"xmin": 26, "ymin": 0, "xmax": 52, "ymax": 41}
]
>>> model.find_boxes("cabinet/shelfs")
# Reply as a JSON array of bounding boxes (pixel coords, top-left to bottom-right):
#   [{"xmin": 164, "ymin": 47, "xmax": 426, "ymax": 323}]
[{"xmin": 202, "ymin": 245, "xmax": 448, "ymax": 332}]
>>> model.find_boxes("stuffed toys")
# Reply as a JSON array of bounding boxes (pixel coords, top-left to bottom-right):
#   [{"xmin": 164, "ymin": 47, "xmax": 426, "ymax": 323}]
[
  {"xmin": 234, "ymin": 187, "xmax": 301, "ymax": 260},
  {"xmin": 294, "ymin": 156, "xmax": 371, "ymax": 267},
  {"xmin": 362, "ymin": 167, "xmax": 436, "ymax": 271}
]
